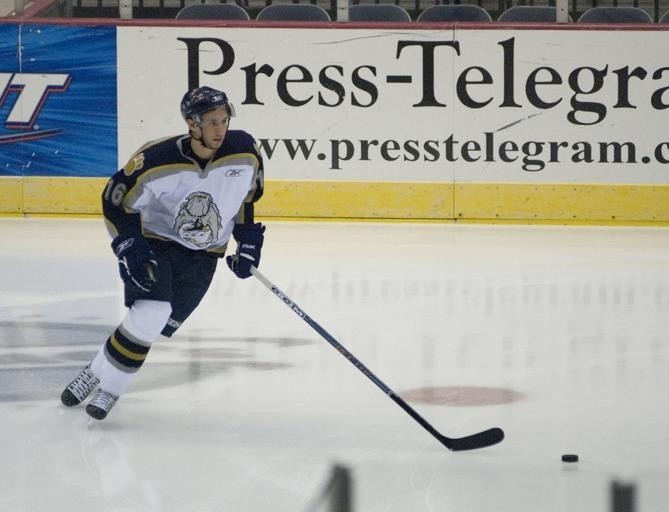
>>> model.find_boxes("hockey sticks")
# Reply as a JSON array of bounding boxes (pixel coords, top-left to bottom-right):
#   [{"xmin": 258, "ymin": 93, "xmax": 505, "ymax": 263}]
[{"xmin": 249, "ymin": 266, "xmax": 505, "ymax": 452}]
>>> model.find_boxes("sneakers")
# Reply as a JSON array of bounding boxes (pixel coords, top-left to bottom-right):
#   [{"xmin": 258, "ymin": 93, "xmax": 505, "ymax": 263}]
[
  {"xmin": 58, "ymin": 360, "xmax": 101, "ymax": 407},
  {"xmin": 85, "ymin": 387, "xmax": 121, "ymax": 421}
]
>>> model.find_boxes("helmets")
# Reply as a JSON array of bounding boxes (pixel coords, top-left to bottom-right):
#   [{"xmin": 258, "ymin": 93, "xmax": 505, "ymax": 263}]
[{"xmin": 180, "ymin": 85, "xmax": 230, "ymax": 125}]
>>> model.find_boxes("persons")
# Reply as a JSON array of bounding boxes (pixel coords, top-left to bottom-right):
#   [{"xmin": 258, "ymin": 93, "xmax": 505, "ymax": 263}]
[{"xmin": 59, "ymin": 86, "xmax": 266, "ymax": 421}]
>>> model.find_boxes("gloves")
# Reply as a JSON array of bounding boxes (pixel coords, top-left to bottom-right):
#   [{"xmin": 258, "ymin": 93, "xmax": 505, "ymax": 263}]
[
  {"xmin": 226, "ymin": 222, "xmax": 267, "ymax": 281},
  {"xmin": 110, "ymin": 227, "xmax": 162, "ymax": 295}
]
